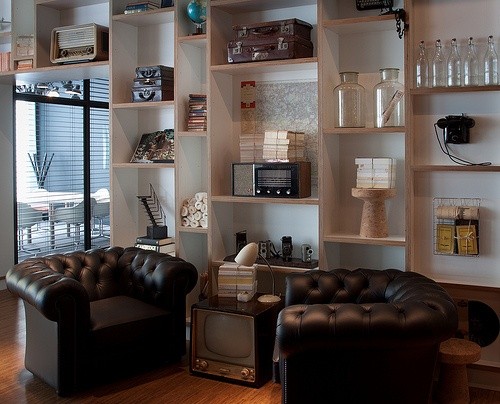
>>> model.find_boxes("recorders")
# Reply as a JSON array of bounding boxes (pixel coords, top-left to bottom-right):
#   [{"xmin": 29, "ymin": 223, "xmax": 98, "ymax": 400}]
[
  {"xmin": 232, "ymin": 162, "xmax": 311, "ymax": 199},
  {"xmin": 50, "ymin": 23, "xmax": 109, "ymax": 64}
]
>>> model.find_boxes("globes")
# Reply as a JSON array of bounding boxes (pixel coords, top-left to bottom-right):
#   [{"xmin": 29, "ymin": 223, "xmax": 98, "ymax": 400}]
[{"xmin": 187, "ymin": 1, "xmax": 206, "ymax": 35}]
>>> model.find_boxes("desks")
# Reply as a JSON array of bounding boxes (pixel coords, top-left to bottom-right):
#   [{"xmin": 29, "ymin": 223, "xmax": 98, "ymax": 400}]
[{"xmin": 18, "ymin": 192, "xmax": 95, "ymax": 249}]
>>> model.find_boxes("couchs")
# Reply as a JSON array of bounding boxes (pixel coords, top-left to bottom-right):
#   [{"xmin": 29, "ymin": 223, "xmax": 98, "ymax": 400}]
[
  {"xmin": 272, "ymin": 269, "xmax": 458, "ymax": 404},
  {"xmin": 5, "ymin": 246, "xmax": 198, "ymax": 397}
]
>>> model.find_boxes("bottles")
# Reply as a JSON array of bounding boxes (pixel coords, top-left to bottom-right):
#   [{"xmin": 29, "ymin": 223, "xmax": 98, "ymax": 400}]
[
  {"xmin": 333, "ymin": 72, "xmax": 365, "ymax": 128},
  {"xmin": 463, "ymin": 36, "xmax": 479, "ymax": 87},
  {"xmin": 415, "ymin": 40, "xmax": 429, "ymax": 88},
  {"xmin": 373, "ymin": 67, "xmax": 405, "ymax": 127},
  {"xmin": 430, "ymin": 39, "xmax": 446, "ymax": 88},
  {"xmin": 484, "ymin": 35, "xmax": 500, "ymax": 85},
  {"xmin": 446, "ymin": 38, "xmax": 462, "ymax": 87}
]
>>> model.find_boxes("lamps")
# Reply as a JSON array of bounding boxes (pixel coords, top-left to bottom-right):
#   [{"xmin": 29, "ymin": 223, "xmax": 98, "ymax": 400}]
[
  {"xmin": 234, "ymin": 243, "xmax": 281, "ymax": 303},
  {"xmin": 16, "ymin": 80, "xmax": 82, "ymax": 100}
]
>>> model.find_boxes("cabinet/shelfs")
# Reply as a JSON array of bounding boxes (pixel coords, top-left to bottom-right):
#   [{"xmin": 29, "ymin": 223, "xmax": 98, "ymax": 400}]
[{"xmin": 0, "ymin": 0, "xmax": 500, "ymax": 325}]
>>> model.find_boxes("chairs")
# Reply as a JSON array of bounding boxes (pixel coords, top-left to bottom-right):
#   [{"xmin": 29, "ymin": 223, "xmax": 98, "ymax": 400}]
[{"xmin": 17, "ymin": 188, "xmax": 110, "ymax": 259}]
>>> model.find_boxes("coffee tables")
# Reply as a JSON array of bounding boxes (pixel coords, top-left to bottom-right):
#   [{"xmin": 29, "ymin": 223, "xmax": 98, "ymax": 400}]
[{"xmin": 188, "ymin": 292, "xmax": 283, "ymax": 390}]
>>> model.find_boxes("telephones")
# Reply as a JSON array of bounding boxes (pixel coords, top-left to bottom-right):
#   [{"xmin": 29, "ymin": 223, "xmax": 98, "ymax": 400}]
[{"xmin": 437, "ymin": 115, "xmax": 476, "ymax": 144}]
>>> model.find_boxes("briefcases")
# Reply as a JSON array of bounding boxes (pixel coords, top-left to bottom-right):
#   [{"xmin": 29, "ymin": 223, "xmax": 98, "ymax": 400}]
[
  {"xmin": 132, "ymin": 66, "xmax": 174, "ymax": 103},
  {"xmin": 226, "ymin": 35, "xmax": 314, "ymax": 63},
  {"xmin": 232, "ymin": 18, "xmax": 313, "ymax": 40}
]
